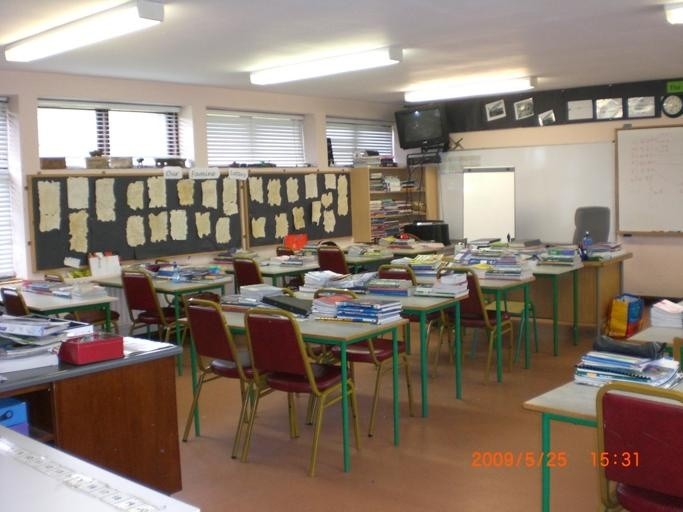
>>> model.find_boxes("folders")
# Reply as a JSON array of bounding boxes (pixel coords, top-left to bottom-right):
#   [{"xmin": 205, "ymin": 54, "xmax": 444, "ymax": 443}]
[{"xmin": 0, "ymin": 320, "xmax": 71, "ymax": 337}]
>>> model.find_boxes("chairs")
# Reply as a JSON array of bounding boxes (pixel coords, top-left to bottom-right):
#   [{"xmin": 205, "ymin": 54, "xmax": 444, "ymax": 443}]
[{"xmin": 574, "ymin": 206, "xmax": 611, "ymax": 245}]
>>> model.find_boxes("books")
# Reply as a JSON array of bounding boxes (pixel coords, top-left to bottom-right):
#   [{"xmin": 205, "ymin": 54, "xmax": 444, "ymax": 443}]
[
  {"xmin": 369, "ymin": 173, "xmax": 426, "ymax": 237},
  {"xmin": 1, "ymin": 240, "xmax": 683, "ymax": 393},
  {"xmin": 353, "ymin": 149, "xmax": 399, "ymax": 167}
]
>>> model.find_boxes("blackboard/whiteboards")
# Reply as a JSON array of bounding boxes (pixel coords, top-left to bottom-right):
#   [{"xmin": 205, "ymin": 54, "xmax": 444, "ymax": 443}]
[
  {"xmin": 244, "ymin": 167, "xmax": 354, "ymax": 249},
  {"xmin": 437, "ymin": 142, "xmax": 617, "ymax": 245},
  {"xmin": 614, "ymin": 124, "xmax": 683, "ymax": 236},
  {"xmin": 27, "ymin": 169, "xmax": 245, "ymax": 275}
]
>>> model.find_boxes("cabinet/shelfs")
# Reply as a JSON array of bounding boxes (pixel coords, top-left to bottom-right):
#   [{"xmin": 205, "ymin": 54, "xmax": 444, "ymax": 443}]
[{"xmin": 351, "ymin": 165, "xmax": 439, "ymax": 243}]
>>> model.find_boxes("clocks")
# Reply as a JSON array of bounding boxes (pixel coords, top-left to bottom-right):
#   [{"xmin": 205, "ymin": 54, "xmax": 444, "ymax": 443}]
[{"xmin": 661, "ymin": 93, "xmax": 683, "ymax": 117}]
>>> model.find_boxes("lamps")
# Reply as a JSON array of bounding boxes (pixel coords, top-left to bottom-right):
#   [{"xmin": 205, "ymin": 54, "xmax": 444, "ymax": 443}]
[
  {"xmin": 248, "ymin": 46, "xmax": 403, "ymax": 88},
  {"xmin": 403, "ymin": 76, "xmax": 537, "ymax": 104},
  {"xmin": 5, "ymin": 0, "xmax": 164, "ymax": 63}
]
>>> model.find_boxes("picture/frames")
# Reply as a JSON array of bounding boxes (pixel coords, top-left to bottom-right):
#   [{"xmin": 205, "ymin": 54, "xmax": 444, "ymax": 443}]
[{"xmin": 484, "ymin": 97, "xmax": 535, "ymax": 122}]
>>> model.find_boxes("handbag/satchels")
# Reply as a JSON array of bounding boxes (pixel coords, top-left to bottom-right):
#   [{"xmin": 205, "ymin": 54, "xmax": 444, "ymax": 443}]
[{"xmin": 607, "ymin": 294, "xmax": 643, "ymax": 338}]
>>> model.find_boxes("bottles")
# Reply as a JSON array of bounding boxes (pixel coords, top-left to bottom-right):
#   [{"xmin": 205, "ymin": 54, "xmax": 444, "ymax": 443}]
[
  {"xmin": 171, "ymin": 261, "xmax": 180, "ymax": 284},
  {"xmin": 582, "ymin": 229, "xmax": 592, "ymax": 261}
]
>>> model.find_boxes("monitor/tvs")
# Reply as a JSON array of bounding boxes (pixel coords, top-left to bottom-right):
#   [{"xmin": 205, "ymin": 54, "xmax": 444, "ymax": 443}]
[{"xmin": 395, "ymin": 102, "xmax": 449, "ymax": 150}]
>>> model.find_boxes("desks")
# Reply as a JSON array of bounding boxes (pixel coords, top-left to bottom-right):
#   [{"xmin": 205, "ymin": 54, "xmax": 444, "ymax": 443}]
[
  {"xmin": 521, "ymin": 327, "xmax": 683, "ymax": 512},
  {"xmin": 485, "ymin": 252, "xmax": 633, "ymax": 356}
]
